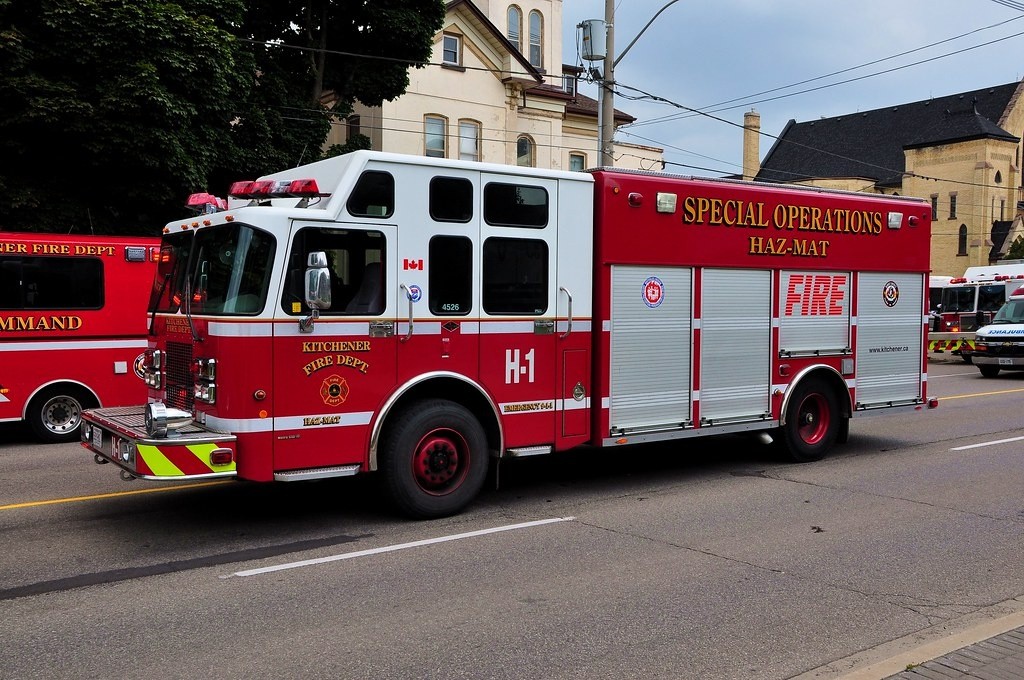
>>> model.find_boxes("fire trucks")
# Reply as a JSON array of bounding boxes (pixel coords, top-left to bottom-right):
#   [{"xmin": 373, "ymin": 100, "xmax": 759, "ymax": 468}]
[
  {"xmin": 924, "ymin": 264, "xmax": 1024, "ymax": 365},
  {"xmin": 76, "ymin": 149, "xmax": 983, "ymax": 519},
  {"xmin": 0, "ymin": 233, "xmax": 174, "ymax": 443}
]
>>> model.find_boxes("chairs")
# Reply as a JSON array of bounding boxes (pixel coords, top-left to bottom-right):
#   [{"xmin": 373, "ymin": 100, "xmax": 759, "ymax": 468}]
[{"xmin": 344, "ymin": 260, "xmax": 383, "ymax": 313}]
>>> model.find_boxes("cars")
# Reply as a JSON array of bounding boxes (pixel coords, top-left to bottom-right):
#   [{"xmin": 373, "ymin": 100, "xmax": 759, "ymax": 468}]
[{"xmin": 974, "ymin": 284, "xmax": 1024, "ymax": 378}]
